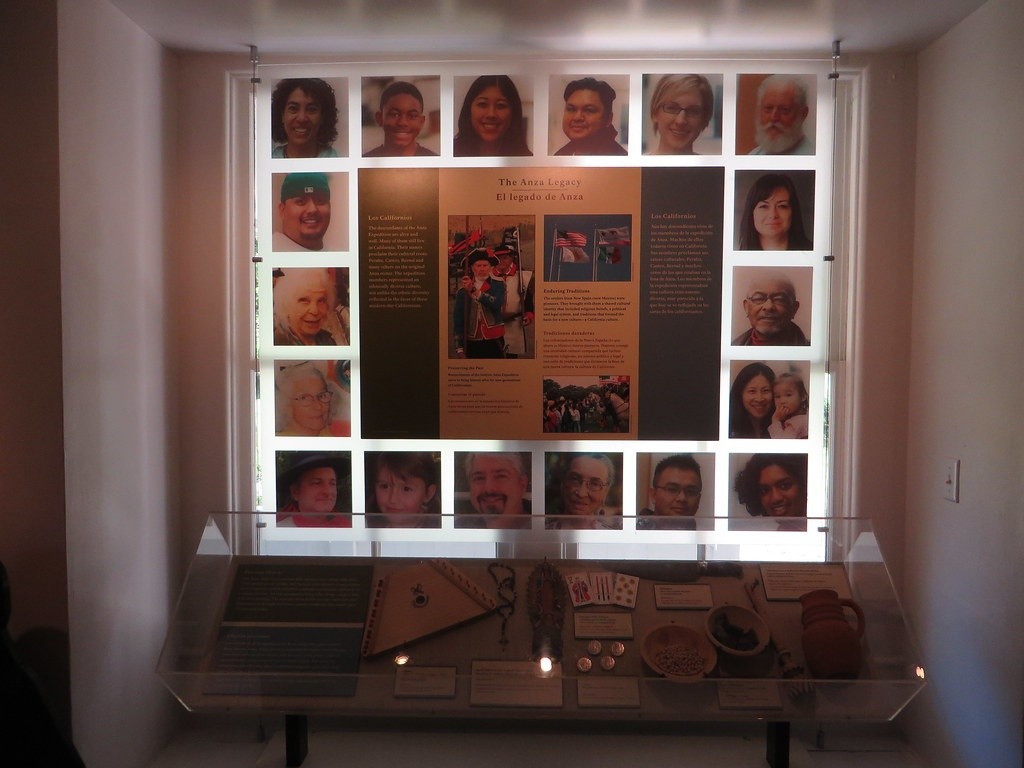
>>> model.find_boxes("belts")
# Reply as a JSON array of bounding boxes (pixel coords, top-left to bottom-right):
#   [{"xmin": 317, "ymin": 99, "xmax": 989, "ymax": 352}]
[{"xmin": 503, "ymin": 311, "xmax": 523, "ymax": 322}]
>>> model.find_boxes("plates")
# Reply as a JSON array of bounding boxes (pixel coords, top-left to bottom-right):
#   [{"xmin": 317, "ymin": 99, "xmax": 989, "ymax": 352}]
[
  {"xmin": 641, "ymin": 623, "xmax": 717, "ymax": 680},
  {"xmin": 704, "ymin": 603, "xmax": 770, "ymax": 658}
]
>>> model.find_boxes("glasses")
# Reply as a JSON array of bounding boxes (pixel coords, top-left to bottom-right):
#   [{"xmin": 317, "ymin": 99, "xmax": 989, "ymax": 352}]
[
  {"xmin": 660, "ymin": 101, "xmax": 708, "ymax": 115},
  {"xmin": 288, "ymin": 391, "xmax": 334, "ymax": 406},
  {"xmin": 748, "ymin": 290, "xmax": 790, "ymax": 306},
  {"xmin": 656, "ymin": 482, "xmax": 700, "ymax": 499},
  {"xmin": 566, "ymin": 474, "xmax": 610, "ymax": 492}
]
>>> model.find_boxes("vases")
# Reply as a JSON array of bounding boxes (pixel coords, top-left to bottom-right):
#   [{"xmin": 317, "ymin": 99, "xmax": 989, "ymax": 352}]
[{"xmin": 799, "ymin": 589, "xmax": 866, "ymax": 679}]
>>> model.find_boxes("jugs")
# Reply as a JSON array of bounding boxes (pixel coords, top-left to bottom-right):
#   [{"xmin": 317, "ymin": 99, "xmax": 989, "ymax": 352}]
[{"xmin": 800, "ymin": 589, "xmax": 866, "ymax": 680}]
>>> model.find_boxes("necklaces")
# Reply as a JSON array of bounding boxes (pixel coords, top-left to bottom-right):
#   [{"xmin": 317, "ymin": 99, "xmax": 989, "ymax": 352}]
[{"xmin": 488, "ymin": 561, "xmax": 517, "ymax": 644}]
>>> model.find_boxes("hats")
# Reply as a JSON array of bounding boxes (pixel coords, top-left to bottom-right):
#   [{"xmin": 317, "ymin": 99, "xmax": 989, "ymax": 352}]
[
  {"xmin": 494, "ymin": 245, "xmax": 514, "ymax": 255},
  {"xmin": 286, "ymin": 453, "xmax": 351, "ymax": 480},
  {"xmin": 460, "ymin": 247, "xmax": 500, "ymax": 267},
  {"xmin": 281, "ymin": 173, "xmax": 330, "ymax": 201}
]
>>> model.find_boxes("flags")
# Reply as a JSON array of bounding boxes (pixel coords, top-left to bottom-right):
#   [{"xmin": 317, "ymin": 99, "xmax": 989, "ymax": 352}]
[{"xmin": 553, "ymin": 224, "xmax": 631, "ymax": 264}]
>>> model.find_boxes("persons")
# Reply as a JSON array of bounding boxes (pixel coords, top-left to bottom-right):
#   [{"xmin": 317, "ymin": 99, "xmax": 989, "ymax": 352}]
[
  {"xmin": 275, "ymin": 361, "xmax": 351, "ymax": 436},
  {"xmin": 732, "ymin": 453, "xmax": 807, "ymax": 532},
  {"xmin": 363, "ymin": 83, "xmax": 438, "ymax": 155},
  {"xmin": 744, "ymin": 72, "xmax": 814, "ymax": 155},
  {"xmin": 731, "ymin": 271, "xmax": 810, "ymax": 346},
  {"xmin": 737, "ymin": 176, "xmax": 811, "ymax": 250},
  {"xmin": 270, "ymin": 78, "xmax": 339, "ymax": 158},
  {"xmin": 545, "ymin": 453, "xmax": 616, "ymax": 528},
  {"xmin": 731, "ymin": 363, "xmax": 808, "ymax": 439},
  {"xmin": 543, "ymin": 383, "xmax": 629, "ymax": 433},
  {"xmin": 555, "ymin": 79, "xmax": 627, "ymax": 155},
  {"xmin": 273, "ymin": 173, "xmax": 332, "ymax": 264},
  {"xmin": 465, "ymin": 452, "xmax": 532, "ymax": 529},
  {"xmin": 454, "ymin": 77, "xmax": 534, "ymax": 155},
  {"xmin": 274, "ymin": 268, "xmax": 350, "ymax": 344},
  {"xmin": 637, "ymin": 454, "xmax": 703, "ymax": 530},
  {"xmin": 455, "ymin": 244, "xmax": 533, "ymax": 359},
  {"xmin": 645, "ymin": 75, "xmax": 713, "ymax": 155},
  {"xmin": 276, "ymin": 452, "xmax": 352, "ymax": 527},
  {"xmin": 372, "ymin": 452, "xmax": 440, "ymax": 527}
]
